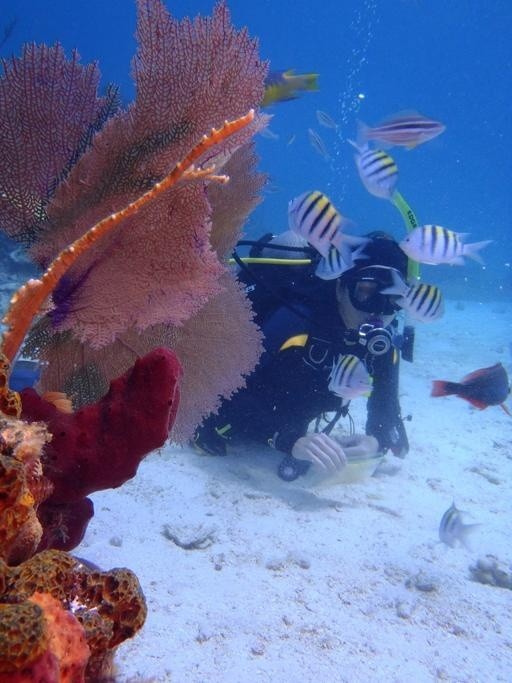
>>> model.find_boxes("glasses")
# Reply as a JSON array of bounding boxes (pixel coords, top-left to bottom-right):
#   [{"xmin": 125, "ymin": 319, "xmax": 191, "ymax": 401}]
[{"xmin": 340, "ymin": 274, "xmax": 408, "ymax": 315}]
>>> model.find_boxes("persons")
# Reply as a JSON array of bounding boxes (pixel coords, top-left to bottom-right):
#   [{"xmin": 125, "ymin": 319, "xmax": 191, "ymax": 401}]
[{"xmin": 180, "ymin": 228, "xmax": 420, "ymax": 477}]
